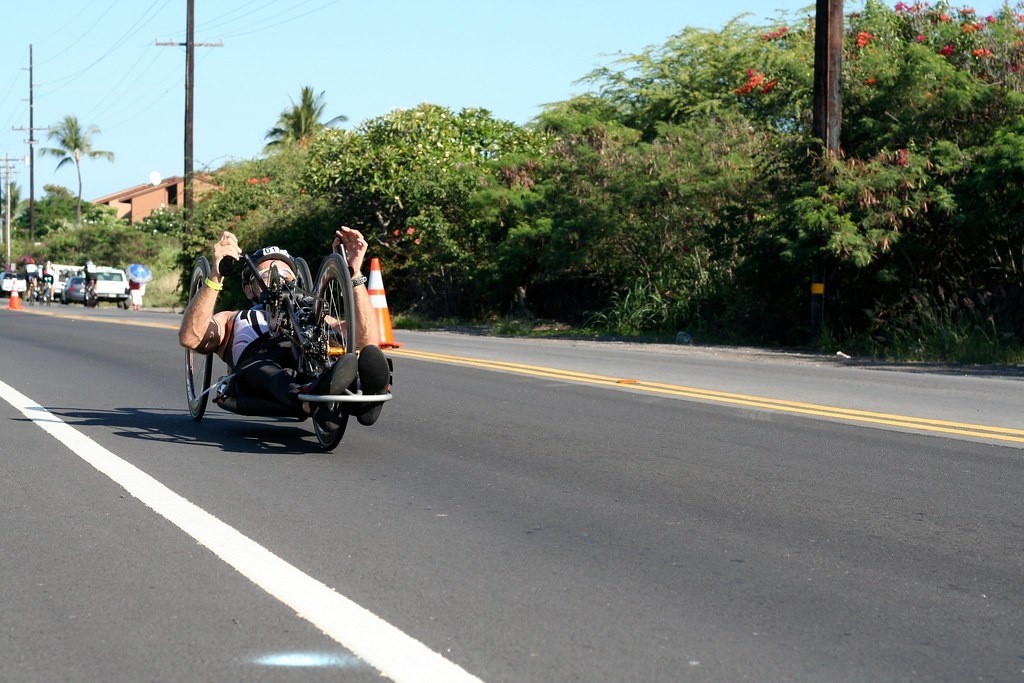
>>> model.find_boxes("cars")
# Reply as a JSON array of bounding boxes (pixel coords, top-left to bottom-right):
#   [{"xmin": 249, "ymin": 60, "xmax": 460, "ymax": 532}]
[{"xmin": 0, "ymin": 262, "xmax": 130, "ymax": 310}]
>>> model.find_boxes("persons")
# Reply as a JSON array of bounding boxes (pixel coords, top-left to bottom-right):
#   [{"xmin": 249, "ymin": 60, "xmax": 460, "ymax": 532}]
[
  {"xmin": 178, "ymin": 226, "xmax": 391, "ymax": 427},
  {"xmin": 23, "ymin": 257, "xmax": 55, "ymax": 301},
  {"xmin": 129, "ymin": 279, "xmax": 143, "ymax": 311},
  {"xmin": 82, "ymin": 262, "xmax": 98, "ymax": 299}
]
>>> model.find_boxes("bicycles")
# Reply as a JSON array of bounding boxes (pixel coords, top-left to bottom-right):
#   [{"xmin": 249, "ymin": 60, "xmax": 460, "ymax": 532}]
[
  {"xmin": 77, "ymin": 269, "xmax": 99, "ymax": 308},
  {"xmin": 28, "ymin": 274, "xmax": 51, "ymax": 308}
]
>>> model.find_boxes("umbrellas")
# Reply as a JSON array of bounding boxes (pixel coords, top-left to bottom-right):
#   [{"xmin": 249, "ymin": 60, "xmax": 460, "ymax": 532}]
[{"xmin": 124, "ymin": 263, "xmax": 153, "ymax": 282}]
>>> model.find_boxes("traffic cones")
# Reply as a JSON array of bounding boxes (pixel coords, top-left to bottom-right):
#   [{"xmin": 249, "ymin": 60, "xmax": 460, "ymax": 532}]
[
  {"xmin": 365, "ymin": 257, "xmax": 403, "ymax": 350},
  {"xmin": 6, "ymin": 281, "xmax": 26, "ymax": 310}
]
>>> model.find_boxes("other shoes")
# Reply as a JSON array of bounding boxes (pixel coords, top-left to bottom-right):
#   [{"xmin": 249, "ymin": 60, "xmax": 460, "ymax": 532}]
[
  {"xmin": 356, "ymin": 345, "xmax": 390, "ymax": 426},
  {"xmin": 308, "ymin": 353, "xmax": 358, "ymax": 417}
]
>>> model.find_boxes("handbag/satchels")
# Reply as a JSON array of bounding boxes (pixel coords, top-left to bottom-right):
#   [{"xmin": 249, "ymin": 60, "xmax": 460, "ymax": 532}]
[{"xmin": 140, "ymin": 284, "xmax": 146, "ymax": 296}]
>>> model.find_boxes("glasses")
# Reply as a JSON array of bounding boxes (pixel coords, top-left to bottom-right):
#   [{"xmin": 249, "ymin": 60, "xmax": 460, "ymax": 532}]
[{"xmin": 251, "ymin": 268, "xmax": 296, "ymax": 283}]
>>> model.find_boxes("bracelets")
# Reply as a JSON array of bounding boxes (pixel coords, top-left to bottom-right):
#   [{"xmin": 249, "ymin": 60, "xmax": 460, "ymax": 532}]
[
  {"xmin": 205, "ymin": 276, "xmax": 223, "ymax": 290},
  {"xmin": 352, "ymin": 276, "xmax": 367, "ymax": 286}
]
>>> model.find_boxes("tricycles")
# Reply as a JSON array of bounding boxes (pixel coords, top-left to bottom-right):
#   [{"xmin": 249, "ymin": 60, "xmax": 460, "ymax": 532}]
[{"xmin": 183, "ymin": 251, "xmax": 388, "ymax": 452}]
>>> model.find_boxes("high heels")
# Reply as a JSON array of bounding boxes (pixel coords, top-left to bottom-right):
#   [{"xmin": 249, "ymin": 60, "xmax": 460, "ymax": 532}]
[{"xmin": 133, "ymin": 306, "xmax": 138, "ymax": 311}]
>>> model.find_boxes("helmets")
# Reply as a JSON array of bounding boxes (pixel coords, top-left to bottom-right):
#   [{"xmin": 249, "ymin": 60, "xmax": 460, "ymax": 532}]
[
  {"xmin": 241, "ymin": 246, "xmax": 298, "ymax": 289},
  {"xmin": 85, "ymin": 262, "xmax": 93, "ymax": 267},
  {"xmin": 29, "ymin": 259, "xmax": 35, "ymax": 263}
]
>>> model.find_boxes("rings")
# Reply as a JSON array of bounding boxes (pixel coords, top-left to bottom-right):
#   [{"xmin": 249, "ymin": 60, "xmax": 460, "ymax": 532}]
[{"xmin": 349, "ymin": 238, "xmax": 352, "ymax": 242}]
